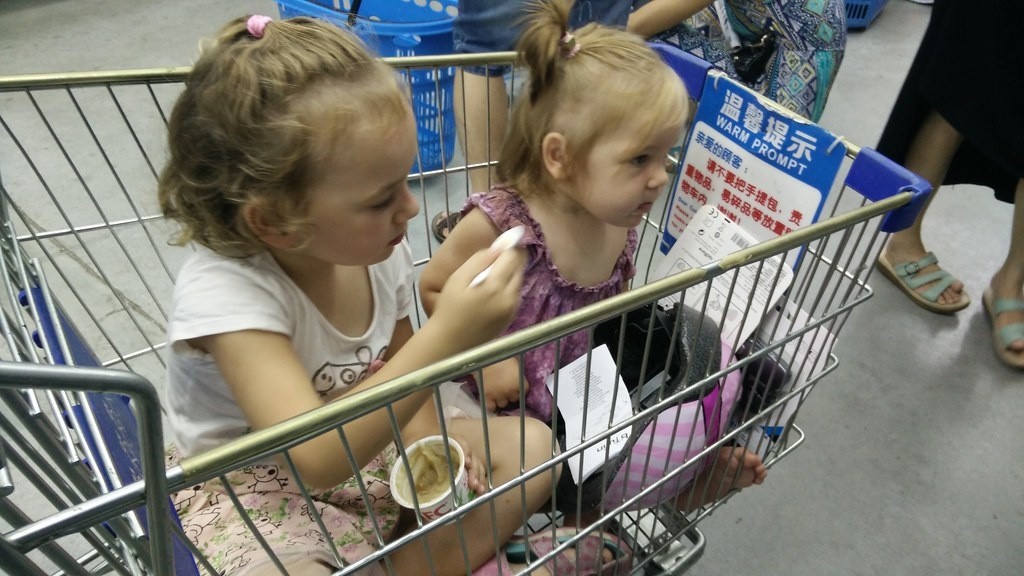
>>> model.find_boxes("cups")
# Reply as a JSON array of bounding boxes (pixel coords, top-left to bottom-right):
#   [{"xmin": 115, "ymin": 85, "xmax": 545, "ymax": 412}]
[{"xmin": 389, "ymin": 435, "xmax": 477, "ymax": 527}]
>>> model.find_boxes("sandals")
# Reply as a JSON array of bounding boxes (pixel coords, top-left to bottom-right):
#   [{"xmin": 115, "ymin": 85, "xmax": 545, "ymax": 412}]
[
  {"xmin": 432, "ymin": 211, "xmax": 463, "ymax": 245},
  {"xmin": 471, "ymin": 527, "xmax": 632, "ymax": 576}
]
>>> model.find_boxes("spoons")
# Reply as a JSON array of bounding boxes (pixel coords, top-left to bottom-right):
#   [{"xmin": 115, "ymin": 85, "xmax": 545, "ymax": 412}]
[{"xmin": 468, "ymin": 225, "xmax": 525, "ymax": 290}]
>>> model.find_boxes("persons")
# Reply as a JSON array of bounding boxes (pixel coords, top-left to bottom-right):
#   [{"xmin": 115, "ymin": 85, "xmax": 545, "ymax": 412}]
[
  {"xmin": 453, "ymin": 1, "xmax": 848, "ymax": 195},
  {"xmin": 418, "ymin": 0, "xmax": 767, "ymax": 530},
  {"xmin": 875, "ymin": 0, "xmax": 1024, "ymax": 367},
  {"xmin": 159, "ymin": 12, "xmax": 562, "ymax": 576}
]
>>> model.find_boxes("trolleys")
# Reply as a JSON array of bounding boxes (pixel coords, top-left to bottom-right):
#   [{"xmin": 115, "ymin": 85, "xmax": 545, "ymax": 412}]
[{"xmin": 0, "ymin": 44, "xmax": 931, "ymax": 576}]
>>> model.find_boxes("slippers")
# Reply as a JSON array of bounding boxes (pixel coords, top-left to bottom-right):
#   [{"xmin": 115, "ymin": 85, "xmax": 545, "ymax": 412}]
[
  {"xmin": 876, "ymin": 249, "xmax": 971, "ymax": 314},
  {"xmin": 983, "ymin": 287, "xmax": 1024, "ymax": 370}
]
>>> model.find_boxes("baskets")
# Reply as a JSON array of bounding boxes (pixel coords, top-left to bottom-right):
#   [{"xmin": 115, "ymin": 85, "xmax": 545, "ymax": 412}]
[
  {"xmin": 278, "ymin": 0, "xmax": 463, "ymax": 177},
  {"xmin": 846, "ymin": 0, "xmax": 891, "ymax": 29}
]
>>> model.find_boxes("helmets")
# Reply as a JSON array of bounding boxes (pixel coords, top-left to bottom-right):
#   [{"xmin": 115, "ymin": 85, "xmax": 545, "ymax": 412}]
[{"xmin": 550, "ymin": 301, "xmax": 743, "ymax": 514}]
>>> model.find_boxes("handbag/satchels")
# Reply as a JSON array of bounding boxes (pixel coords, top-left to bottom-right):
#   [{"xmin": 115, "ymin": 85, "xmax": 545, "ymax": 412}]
[{"xmin": 732, "ymin": 33, "xmax": 777, "ymax": 85}]
